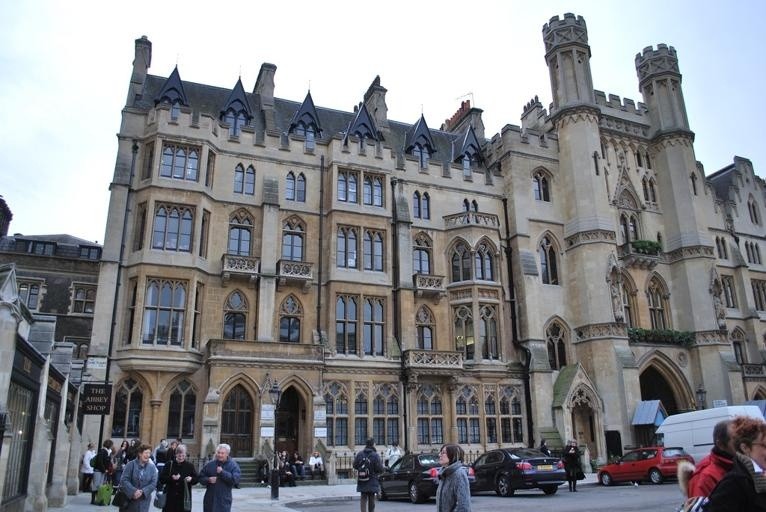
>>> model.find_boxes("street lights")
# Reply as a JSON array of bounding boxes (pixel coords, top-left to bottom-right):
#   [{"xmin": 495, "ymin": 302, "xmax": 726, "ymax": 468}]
[{"xmin": 696, "ymin": 383, "xmax": 707, "ymax": 409}]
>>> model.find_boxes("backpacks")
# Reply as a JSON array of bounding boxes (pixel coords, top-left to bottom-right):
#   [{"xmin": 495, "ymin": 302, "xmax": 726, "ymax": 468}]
[{"xmin": 358, "ymin": 453, "xmax": 371, "ymax": 482}]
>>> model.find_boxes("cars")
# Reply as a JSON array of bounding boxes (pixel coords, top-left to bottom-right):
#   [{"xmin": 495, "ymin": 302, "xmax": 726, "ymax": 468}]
[
  {"xmin": 597, "ymin": 446, "xmax": 697, "ymax": 485},
  {"xmin": 472, "ymin": 447, "xmax": 566, "ymax": 495},
  {"xmin": 375, "ymin": 453, "xmax": 476, "ymax": 503}
]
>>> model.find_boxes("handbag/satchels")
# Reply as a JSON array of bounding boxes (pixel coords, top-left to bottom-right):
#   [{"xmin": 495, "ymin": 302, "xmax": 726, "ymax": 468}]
[
  {"xmin": 94, "ymin": 483, "xmax": 112, "ymax": 506},
  {"xmin": 676, "ymin": 496, "xmax": 710, "ymax": 512},
  {"xmin": 112, "ymin": 492, "xmax": 129, "ymax": 508},
  {"xmin": 153, "ymin": 492, "xmax": 167, "ymax": 508},
  {"xmin": 90, "ymin": 455, "xmax": 97, "ymax": 466}
]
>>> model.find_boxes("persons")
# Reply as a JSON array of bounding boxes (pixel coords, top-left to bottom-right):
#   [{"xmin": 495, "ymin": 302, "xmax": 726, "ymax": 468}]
[
  {"xmin": 436, "ymin": 444, "xmax": 471, "ymax": 512},
  {"xmin": 563, "ymin": 439, "xmax": 581, "ymax": 492},
  {"xmin": 384, "ymin": 442, "xmax": 405, "ymax": 469},
  {"xmin": 676, "ymin": 416, "xmax": 766, "ymax": 511},
  {"xmin": 539, "ymin": 439, "xmax": 551, "ymax": 457},
  {"xmin": 562, "ymin": 440, "xmax": 571, "ymax": 458},
  {"xmin": 79, "ymin": 437, "xmax": 240, "ymax": 512},
  {"xmin": 353, "ymin": 439, "xmax": 385, "ymax": 512},
  {"xmin": 256, "ymin": 450, "xmax": 325, "ymax": 486}
]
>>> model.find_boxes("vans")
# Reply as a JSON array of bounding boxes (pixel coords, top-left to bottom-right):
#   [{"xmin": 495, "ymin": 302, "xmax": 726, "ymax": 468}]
[{"xmin": 652, "ymin": 404, "xmax": 766, "ymax": 473}]
